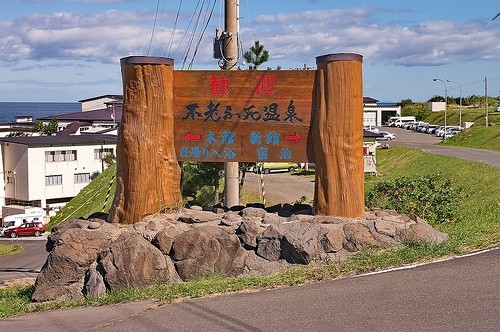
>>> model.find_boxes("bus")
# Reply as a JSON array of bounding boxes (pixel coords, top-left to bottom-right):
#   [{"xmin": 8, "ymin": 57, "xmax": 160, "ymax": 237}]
[{"xmin": 388, "ymin": 116, "xmax": 416, "ymax": 126}]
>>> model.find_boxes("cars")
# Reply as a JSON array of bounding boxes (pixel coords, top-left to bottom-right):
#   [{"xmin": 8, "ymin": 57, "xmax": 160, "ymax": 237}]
[
  {"xmin": 366, "ymin": 127, "xmax": 379, "ymax": 134},
  {"xmin": 376, "ymin": 132, "xmax": 395, "ymax": 140},
  {"xmin": 494, "ymin": 105, "xmax": 500, "ymax": 111},
  {"xmin": 403, "ymin": 121, "xmax": 464, "ymax": 138}
]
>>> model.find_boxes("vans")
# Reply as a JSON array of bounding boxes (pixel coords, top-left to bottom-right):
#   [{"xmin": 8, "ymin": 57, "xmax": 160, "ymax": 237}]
[
  {"xmin": 395, "ymin": 119, "xmax": 415, "ymax": 127},
  {"xmin": 1, "ymin": 214, "xmax": 44, "ymax": 236}
]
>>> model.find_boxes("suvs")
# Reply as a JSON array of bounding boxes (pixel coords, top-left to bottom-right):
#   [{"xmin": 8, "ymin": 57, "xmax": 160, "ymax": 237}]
[{"xmin": 4, "ymin": 222, "xmax": 46, "ymax": 238}]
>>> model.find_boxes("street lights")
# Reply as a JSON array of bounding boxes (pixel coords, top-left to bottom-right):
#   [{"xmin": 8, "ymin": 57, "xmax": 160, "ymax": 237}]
[
  {"xmin": 446, "ymin": 80, "xmax": 462, "ymax": 127},
  {"xmin": 433, "ymin": 78, "xmax": 448, "ymax": 140}
]
